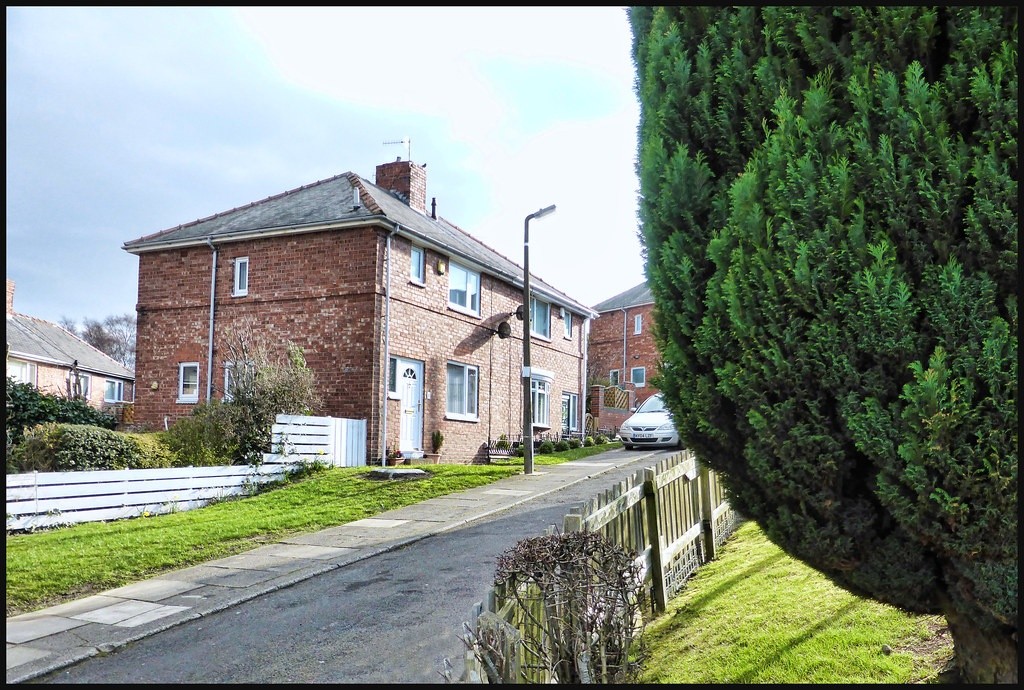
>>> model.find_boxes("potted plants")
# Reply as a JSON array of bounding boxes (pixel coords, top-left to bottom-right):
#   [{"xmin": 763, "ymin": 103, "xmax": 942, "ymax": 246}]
[{"xmin": 426, "ymin": 430, "xmax": 444, "ymax": 464}]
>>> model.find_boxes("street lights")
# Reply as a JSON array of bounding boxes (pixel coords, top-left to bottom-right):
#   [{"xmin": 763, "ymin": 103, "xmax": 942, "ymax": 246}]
[{"xmin": 523, "ymin": 204, "xmax": 556, "ymax": 474}]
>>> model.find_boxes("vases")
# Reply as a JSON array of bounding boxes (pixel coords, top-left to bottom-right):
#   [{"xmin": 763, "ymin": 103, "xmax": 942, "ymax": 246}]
[{"xmin": 388, "ymin": 458, "xmax": 405, "ymax": 465}]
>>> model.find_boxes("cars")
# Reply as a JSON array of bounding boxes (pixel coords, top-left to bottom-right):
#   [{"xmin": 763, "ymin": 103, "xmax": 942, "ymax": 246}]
[{"xmin": 619, "ymin": 393, "xmax": 688, "ymax": 450}]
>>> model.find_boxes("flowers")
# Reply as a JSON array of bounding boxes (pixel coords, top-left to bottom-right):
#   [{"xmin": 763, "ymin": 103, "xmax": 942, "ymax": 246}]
[{"xmin": 390, "ymin": 450, "xmax": 404, "ymax": 458}]
